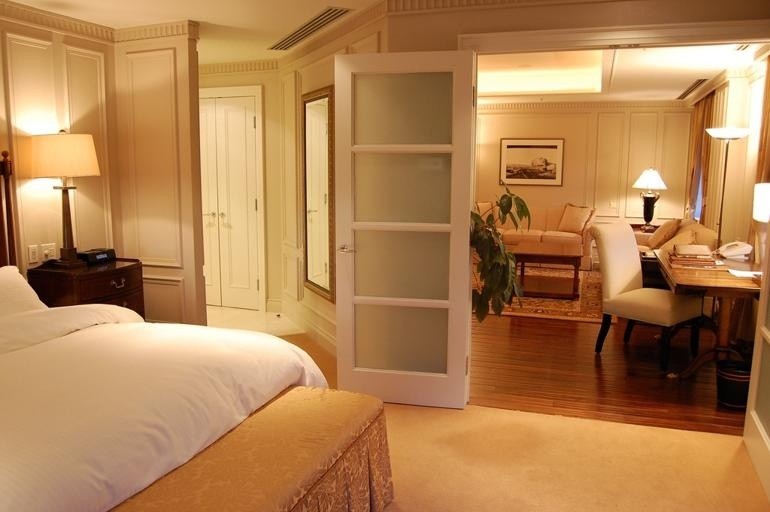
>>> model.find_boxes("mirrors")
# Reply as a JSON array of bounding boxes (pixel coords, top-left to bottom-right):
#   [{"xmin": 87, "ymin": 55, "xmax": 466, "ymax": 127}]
[{"xmin": 302, "ymin": 84, "xmax": 335, "ymax": 304}]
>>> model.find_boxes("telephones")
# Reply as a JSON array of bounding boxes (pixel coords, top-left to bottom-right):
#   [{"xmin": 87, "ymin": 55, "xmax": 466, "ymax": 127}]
[{"xmin": 718, "ymin": 240, "xmax": 753, "ymax": 258}]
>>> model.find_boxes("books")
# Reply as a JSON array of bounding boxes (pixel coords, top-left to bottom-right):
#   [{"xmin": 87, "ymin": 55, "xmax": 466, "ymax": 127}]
[{"xmin": 666, "ymin": 241, "xmax": 717, "ymax": 271}]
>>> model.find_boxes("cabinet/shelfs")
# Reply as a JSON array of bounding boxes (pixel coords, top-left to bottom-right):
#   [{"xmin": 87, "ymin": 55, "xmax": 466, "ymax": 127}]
[
  {"xmin": 27, "ymin": 258, "xmax": 145, "ymax": 322},
  {"xmin": 305, "ymin": 95, "xmax": 328, "ymax": 290},
  {"xmin": 200, "ymin": 85, "xmax": 266, "ymax": 312}
]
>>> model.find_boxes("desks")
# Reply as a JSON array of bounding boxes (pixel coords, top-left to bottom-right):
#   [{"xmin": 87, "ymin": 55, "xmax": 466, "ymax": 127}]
[
  {"xmin": 509, "ymin": 239, "xmax": 582, "ymax": 300},
  {"xmin": 653, "ymin": 248, "xmax": 764, "ymax": 387}
]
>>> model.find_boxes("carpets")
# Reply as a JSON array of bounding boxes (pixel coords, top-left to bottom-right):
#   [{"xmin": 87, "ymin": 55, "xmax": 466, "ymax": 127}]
[
  {"xmin": 470, "ymin": 248, "xmax": 618, "ymax": 325},
  {"xmin": 206, "ymin": 305, "xmax": 305, "ymax": 337}
]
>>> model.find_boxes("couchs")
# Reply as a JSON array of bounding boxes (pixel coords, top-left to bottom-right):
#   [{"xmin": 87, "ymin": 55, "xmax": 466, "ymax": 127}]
[
  {"xmin": 477, "ymin": 202, "xmax": 597, "ymax": 258},
  {"xmin": 632, "ymin": 219, "xmax": 719, "ymax": 252}
]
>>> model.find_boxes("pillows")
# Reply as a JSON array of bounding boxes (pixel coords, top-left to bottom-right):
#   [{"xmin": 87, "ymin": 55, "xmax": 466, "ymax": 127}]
[
  {"xmin": 0, "ymin": 266, "xmax": 49, "ymax": 317},
  {"xmin": 648, "ymin": 216, "xmax": 698, "ymax": 248},
  {"xmin": 477, "ymin": 202, "xmax": 493, "ymax": 227},
  {"xmin": 557, "ymin": 203, "xmax": 596, "ymax": 235}
]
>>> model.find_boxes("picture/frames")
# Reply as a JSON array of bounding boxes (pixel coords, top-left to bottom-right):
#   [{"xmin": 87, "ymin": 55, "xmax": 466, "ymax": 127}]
[{"xmin": 498, "ymin": 138, "xmax": 565, "ymax": 187}]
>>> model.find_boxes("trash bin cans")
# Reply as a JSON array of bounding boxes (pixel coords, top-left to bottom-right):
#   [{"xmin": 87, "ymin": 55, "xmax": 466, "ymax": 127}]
[{"xmin": 716, "ymin": 359, "xmax": 752, "ymax": 412}]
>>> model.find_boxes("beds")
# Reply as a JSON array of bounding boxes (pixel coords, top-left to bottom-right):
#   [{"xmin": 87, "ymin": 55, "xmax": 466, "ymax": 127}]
[{"xmin": 0, "ymin": 266, "xmax": 395, "ymax": 512}]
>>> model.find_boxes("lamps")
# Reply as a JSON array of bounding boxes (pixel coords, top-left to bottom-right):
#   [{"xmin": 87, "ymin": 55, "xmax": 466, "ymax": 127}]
[
  {"xmin": 632, "ymin": 169, "xmax": 667, "ymax": 232},
  {"xmin": 705, "ymin": 128, "xmax": 753, "ymax": 248},
  {"xmin": 28, "ymin": 129, "xmax": 100, "ymax": 269}
]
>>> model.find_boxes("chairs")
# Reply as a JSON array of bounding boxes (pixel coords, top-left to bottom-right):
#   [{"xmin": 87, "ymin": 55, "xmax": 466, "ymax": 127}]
[{"xmin": 591, "ymin": 220, "xmax": 703, "ymax": 372}]
